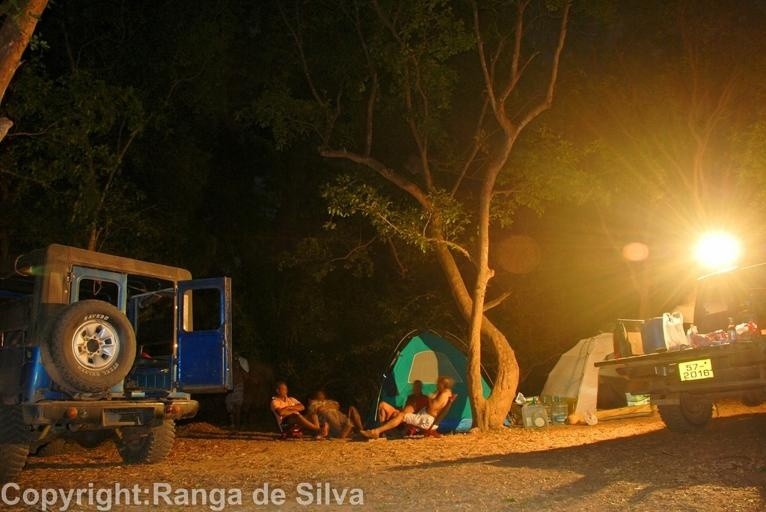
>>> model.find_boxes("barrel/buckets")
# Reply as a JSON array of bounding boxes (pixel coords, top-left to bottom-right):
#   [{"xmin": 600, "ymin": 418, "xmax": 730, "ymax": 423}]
[{"xmin": 521, "ymin": 394, "xmax": 569, "ymax": 427}]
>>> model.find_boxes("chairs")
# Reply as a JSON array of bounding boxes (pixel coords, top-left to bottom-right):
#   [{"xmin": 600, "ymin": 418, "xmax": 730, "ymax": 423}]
[{"xmin": 269, "ymin": 391, "xmax": 458, "ymax": 441}]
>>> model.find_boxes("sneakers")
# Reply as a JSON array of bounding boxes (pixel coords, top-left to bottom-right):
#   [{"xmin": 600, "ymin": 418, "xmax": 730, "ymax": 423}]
[{"xmin": 312, "ymin": 429, "xmax": 404, "ymax": 440}]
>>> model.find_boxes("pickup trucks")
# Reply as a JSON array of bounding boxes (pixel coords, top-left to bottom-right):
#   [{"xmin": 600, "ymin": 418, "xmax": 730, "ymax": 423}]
[{"xmin": 595, "ymin": 261, "xmax": 766, "ymax": 432}]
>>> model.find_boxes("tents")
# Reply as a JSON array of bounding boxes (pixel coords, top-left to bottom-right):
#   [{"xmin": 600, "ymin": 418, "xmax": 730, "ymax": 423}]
[
  {"xmin": 365, "ymin": 325, "xmax": 515, "ymax": 430},
  {"xmin": 537, "ymin": 326, "xmax": 644, "ymax": 419}
]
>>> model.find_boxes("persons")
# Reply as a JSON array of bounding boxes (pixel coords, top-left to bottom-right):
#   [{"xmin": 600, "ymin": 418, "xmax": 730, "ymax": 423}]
[
  {"xmin": 303, "ymin": 389, "xmax": 351, "ymax": 439},
  {"xmin": 379, "ymin": 378, "xmax": 429, "ymax": 425},
  {"xmin": 358, "ymin": 374, "xmax": 455, "ymax": 439},
  {"xmin": 270, "ymin": 379, "xmax": 328, "ymax": 441}
]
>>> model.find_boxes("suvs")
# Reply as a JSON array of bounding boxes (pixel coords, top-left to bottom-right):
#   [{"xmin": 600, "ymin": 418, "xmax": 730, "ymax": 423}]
[{"xmin": 0, "ymin": 243, "xmax": 234, "ymax": 482}]
